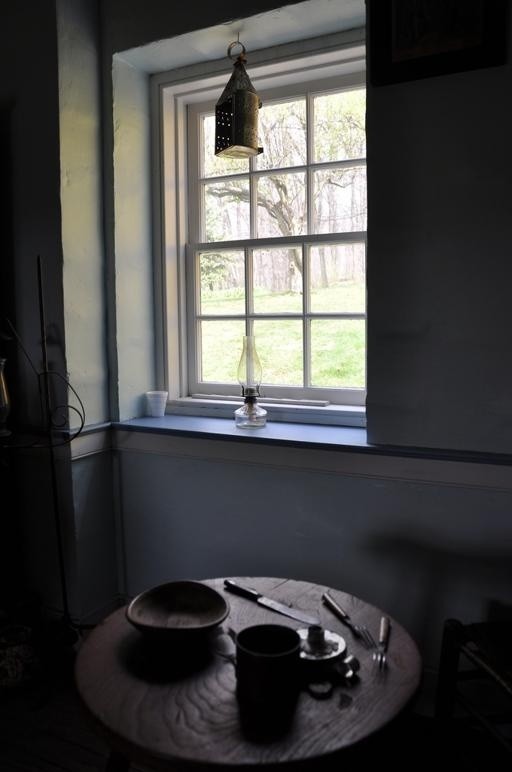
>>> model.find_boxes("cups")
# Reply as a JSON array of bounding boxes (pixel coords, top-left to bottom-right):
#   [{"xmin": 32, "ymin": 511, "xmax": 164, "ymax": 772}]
[
  {"xmin": 232, "ymin": 625, "xmax": 300, "ymax": 705},
  {"xmin": 146, "ymin": 390, "xmax": 170, "ymax": 417}
]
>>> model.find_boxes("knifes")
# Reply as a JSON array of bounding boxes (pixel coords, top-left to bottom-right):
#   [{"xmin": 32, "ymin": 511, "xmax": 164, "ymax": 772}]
[{"xmin": 222, "ymin": 579, "xmax": 322, "ymax": 627}]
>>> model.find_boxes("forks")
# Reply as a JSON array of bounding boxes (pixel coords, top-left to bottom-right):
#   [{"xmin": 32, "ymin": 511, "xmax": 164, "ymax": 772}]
[
  {"xmin": 324, "ymin": 592, "xmax": 375, "ymax": 649},
  {"xmin": 371, "ymin": 619, "xmax": 390, "ymax": 674}
]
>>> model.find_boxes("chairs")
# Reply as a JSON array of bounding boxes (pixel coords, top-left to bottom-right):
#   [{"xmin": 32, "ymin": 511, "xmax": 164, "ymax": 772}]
[{"xmin": 440, "ymin": 600, "xmax": 511, "ymax": 772}]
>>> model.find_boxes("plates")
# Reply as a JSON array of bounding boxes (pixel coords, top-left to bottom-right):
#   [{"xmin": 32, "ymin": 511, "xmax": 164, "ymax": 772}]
[
  {"xmin": 125, "ymin": 579, "xmax": 233, "ymax": 646},
  {"xmin": 293, "ymin": 626, "xmax": 346, "ymax": 661}
]
